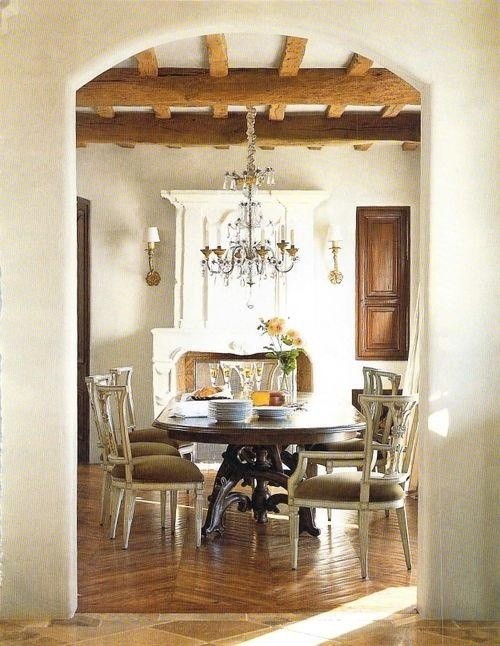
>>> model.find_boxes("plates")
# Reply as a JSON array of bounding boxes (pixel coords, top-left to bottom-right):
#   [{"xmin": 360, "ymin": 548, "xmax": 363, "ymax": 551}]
[{"xmin": 207, "ymin": 400, "xmax": 288, "ymax": 425}]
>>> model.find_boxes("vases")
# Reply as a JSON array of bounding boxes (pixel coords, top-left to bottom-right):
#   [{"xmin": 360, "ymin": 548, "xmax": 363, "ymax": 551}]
[{"xmin": 278, "ymin": 371, "xmax": 292, "ymax": 396}]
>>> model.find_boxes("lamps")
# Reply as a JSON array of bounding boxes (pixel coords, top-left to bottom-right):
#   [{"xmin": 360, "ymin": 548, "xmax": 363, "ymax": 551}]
[
  {"xmin": 327, "ymin": 222, "xmax": 344, "ymax": 284},
  {"xmin": 199, "ymin": 104, "xmax": 301, "ymax": 287},
  {"xmin": 141, "ymin": 226, "xmax": 161, "ymax": 287}
]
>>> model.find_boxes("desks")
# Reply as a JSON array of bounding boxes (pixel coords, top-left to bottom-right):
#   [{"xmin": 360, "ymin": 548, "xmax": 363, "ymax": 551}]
[{"xmin": 151, "ymin": 391, "xmax": 367, "ymax": 539}]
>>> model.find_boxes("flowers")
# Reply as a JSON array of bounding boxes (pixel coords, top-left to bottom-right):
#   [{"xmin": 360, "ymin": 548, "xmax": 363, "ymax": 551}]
[{"xmin": 256, "ymin": 316, "xmax": 303, "ymax": 390}]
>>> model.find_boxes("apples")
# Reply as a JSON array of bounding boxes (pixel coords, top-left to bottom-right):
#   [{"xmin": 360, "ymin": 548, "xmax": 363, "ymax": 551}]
[{"xmin": 270, "ymin": 392, "xmax": 285, "ymax": 406}]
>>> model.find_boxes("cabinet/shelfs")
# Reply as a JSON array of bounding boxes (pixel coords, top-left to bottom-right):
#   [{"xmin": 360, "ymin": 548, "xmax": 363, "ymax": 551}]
[{"xmin": 355, "ymin": 205, "xmax": 412, "ymax": 362}]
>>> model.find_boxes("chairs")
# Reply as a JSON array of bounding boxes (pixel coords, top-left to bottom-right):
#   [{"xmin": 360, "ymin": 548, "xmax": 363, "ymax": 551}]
[
  {"xmin": 285, "ymin": 361, "xmax": 420, "ymax": 580},
  {"xmin": 85, "ymin": 365, "xmax": 204, "ymax": 555}
]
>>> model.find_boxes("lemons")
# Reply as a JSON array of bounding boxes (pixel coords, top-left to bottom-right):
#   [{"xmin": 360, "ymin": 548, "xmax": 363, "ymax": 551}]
[{"xmin": 252, "ymin": 390, "xmax": 270, "ymax": 406}]
